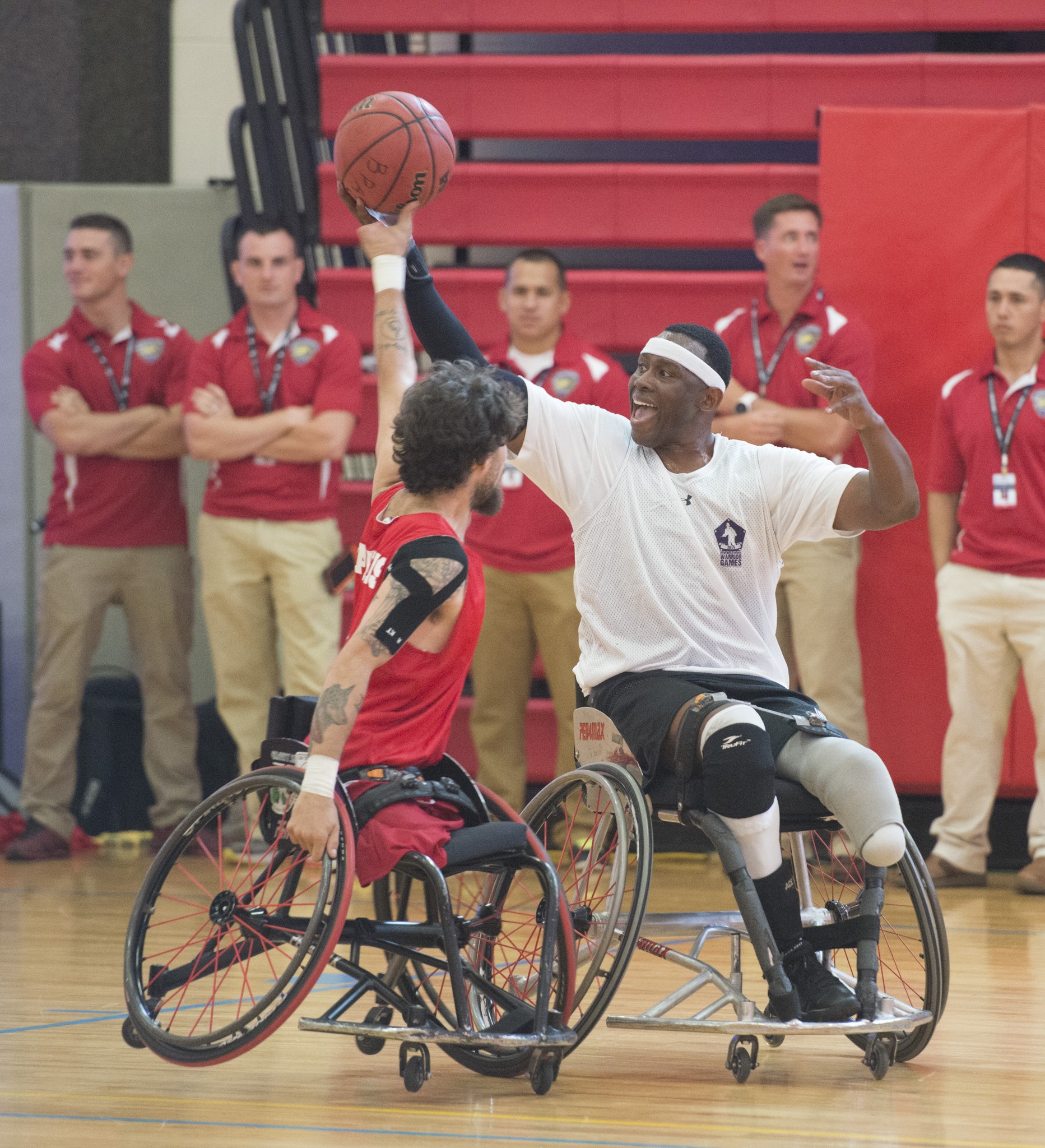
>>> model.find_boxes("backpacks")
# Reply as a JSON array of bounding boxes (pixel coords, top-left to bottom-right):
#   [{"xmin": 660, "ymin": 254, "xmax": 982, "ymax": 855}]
[{"xmin": 71, "ymin": 665, "xmax": 154, "ymax": 836}]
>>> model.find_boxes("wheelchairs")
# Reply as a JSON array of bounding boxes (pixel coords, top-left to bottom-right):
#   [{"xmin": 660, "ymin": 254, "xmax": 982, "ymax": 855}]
[
  {"xmin": 116, "ymin": 685, "xmax": 579, "ymax": 1097},
  {"xmin": 463, "ymin": 662, "xmax": 952, "ymax": 1085}
]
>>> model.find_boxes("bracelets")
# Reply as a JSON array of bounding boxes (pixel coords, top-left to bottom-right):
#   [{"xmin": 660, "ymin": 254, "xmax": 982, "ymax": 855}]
[
  {"xmin": 372, "ymin": 254, "xmax": 406, "ymax": 291},
  {"xmin": 299, "ymin": 753, "xmax": 339, "ymax": 799}
]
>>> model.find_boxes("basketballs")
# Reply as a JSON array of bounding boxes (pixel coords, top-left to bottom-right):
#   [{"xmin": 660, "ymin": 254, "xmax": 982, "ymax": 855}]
[{"xmin": 333, "ymin": 91, "xmax": 456, "ymax": 215}]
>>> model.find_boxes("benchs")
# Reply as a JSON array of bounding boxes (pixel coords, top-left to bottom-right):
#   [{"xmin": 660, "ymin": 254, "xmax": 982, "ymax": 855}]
[{"xmin": 295, "ymin": 0, "xmax": 1045, "ymax": 809}]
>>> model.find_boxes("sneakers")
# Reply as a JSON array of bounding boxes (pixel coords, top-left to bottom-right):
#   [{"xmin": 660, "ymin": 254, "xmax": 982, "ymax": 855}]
[
  {"xmin": 147, "ymin": 815, "xmax": 223, "ymax": 861},
  {"xmin": 768, "ymin": 940, "xmax": 863, "ymax": 1022},
  {"xmin": 7, "ymin": 816, "xmax": 72, "ymax": 860}
]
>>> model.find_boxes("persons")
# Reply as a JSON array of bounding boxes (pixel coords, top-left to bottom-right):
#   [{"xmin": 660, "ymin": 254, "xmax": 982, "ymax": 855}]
[
  {"xmin": 714, "ymin": 194, "xmax": 888, "ymax": 883},
  {"xmin": 893, "ymin": 251, "xmax": 1044, "ymax": 895},
  {"xmin": 1, "ymin": 213, "xmax": 365, "ymax": 868},
  {"xmin": 452, "ymin": 253, "xmax": 628, "ymax": 861},
  {"xmin": 272, "ymin": 198, "xmax": 528, "ymax": 866},
  {"xmin": 336, "ymin": 174, "xmax": 924, "ymax": 1020}
]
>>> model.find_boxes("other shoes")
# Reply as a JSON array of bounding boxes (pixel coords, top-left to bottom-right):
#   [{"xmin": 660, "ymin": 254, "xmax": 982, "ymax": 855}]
[
  {"xmin": 223, "ymin": 836, "xmax": 279, "ymax": 865},
  {"xmin": 828, "ymin": 856, "xmax": 866, "ymax": 883},
  {"xmin": 898, "ymin": 854, "xmax": 988, "ymax": 888},
  {"xmin": 1013, "ymin": 855, "xmax": 1045, "ymax": 895},
  {"xmin": 570, "ymin": 837, "xmax": 610, "ymax": 872}
]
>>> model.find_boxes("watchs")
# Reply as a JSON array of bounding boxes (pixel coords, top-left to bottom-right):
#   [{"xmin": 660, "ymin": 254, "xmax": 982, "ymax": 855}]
[{"xmin": 735, "ymin": 387, "xmax": 757, "ymax": 413}]
